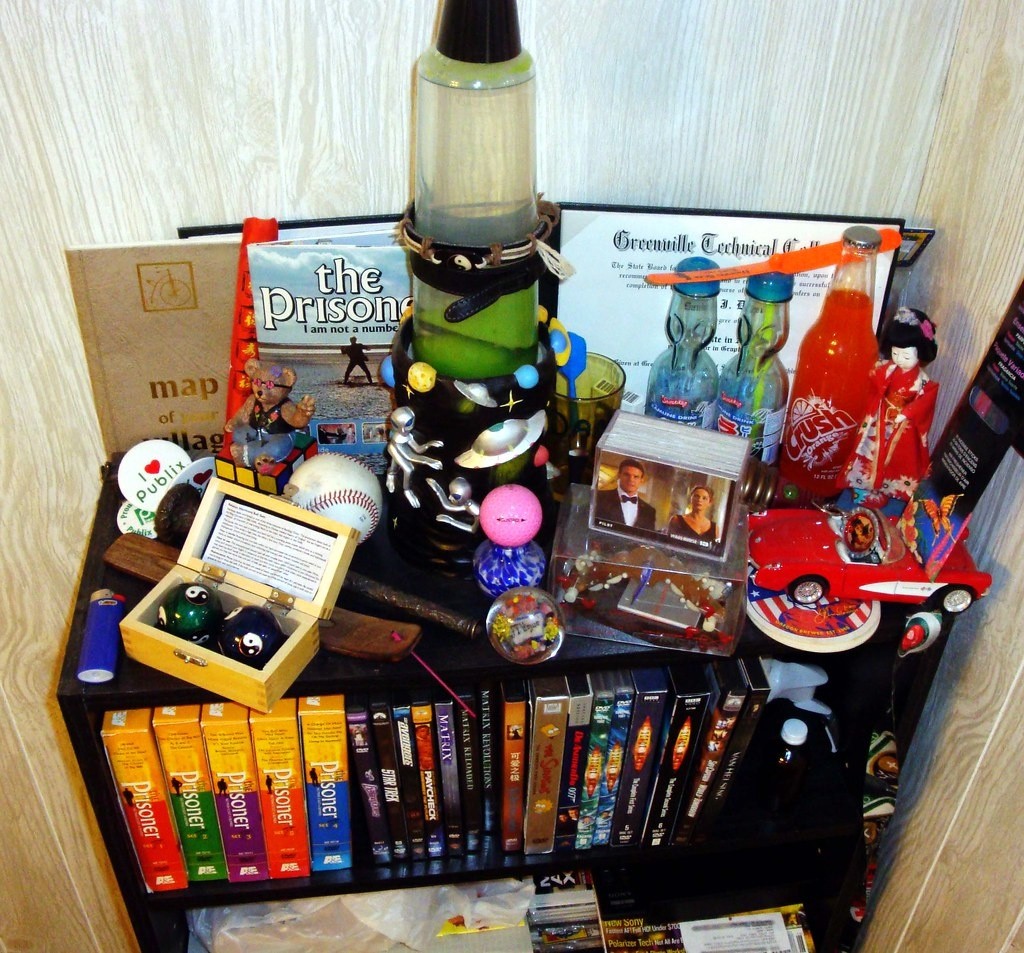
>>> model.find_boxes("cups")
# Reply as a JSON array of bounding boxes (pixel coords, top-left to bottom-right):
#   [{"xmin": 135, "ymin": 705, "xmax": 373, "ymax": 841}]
[{"xmin": 552, "ymin": 353, "xmax": 626, "ymax": 485}]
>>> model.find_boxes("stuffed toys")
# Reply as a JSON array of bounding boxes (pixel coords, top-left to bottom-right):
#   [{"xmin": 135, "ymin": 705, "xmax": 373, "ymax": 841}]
[{"xmin": 223, "ymin": 360, "xmax": 317, "ymax": 476}]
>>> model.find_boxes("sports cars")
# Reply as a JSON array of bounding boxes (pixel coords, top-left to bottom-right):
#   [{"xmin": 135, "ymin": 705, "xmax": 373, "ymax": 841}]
[{"xmin": 735, "ymin": 503, "xmax": 992, "ymax": 617}]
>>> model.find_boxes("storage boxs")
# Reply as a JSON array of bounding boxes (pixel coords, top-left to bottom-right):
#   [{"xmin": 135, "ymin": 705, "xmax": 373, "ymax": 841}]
[{"xmin": 120, "ymin": 474, "xmax": 360, "ymax": 715}]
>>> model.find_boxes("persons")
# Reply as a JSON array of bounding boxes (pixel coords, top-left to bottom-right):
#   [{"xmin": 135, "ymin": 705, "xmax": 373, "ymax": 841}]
[
  {"xmin": 838, "ymin": 311, "xmax": 939, "ymax": 508},
  {"xmin": 332, "ymin": 336, "xmax": 374, "ymax": 385},
  {"xmin": 668, "ymin": 485, "xmax": 719, "ymax": 541},
  {"xmin": 596, "ymin": 459, "xmax": 657, "ymax": 531},
  {"xmin": 388, "ymin": 408, "xmax": 444, "ymax": 507},
  {"xmin": 427, "ymin": 477, "xmax": 480, "ymax": 535},
  {"xmin": 319, "ymin": 424, "xmax": 355, "ymax": 445},
  {"xmin": 367, "ymin": 427, "xmax": 385, "ymax": 442}
]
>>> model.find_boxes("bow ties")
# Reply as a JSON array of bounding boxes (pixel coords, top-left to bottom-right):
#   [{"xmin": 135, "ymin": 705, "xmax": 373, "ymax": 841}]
[{"xmin": 621, "ymin": 494, "xmax": 637, "ymax": 504}]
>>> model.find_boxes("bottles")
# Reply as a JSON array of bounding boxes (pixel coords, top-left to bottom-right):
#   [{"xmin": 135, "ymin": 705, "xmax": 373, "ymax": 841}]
[
  {"xmin": 409, "ymin": 0, "xmax": 536, "ymax": 375},
  {"xmin": 713, "ymin": 267, "xmax": 792, "ymax": 468},
  {"xmin": 643, "ymin": 258, "xmax": 718, "ymax": 433},
  {"xmin": 783, "ymin": 226, "xmax": 880, "ymax": 499}
]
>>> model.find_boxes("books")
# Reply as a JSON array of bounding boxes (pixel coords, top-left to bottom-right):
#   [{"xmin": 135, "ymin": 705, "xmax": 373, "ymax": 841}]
[{"xmin": 616, "ymin": 575, "xmax": 704, "ymax": 629}]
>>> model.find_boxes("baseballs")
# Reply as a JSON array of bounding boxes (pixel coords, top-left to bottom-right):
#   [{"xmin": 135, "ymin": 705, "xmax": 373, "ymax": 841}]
[{"xmin": 280, "ymin": 453, "xmax": 380, "ymax": 547}]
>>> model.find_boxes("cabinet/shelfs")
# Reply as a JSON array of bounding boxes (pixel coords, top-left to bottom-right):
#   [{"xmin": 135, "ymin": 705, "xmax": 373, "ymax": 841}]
[{"xmin": 58, "ymin": 453, "xmax": 957, "ymax": 953}]
